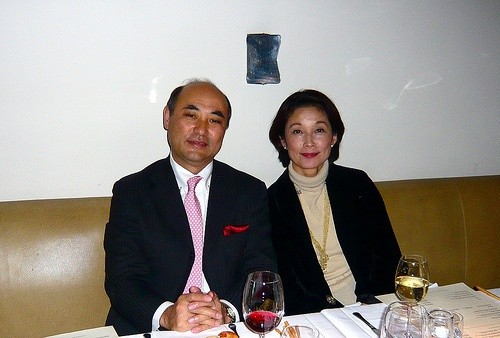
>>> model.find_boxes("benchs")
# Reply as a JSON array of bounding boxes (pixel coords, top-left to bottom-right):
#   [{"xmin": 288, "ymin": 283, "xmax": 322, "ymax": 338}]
[{"xmin": 0, "ymin": 175, "xmax": 500, "ymax": 338}]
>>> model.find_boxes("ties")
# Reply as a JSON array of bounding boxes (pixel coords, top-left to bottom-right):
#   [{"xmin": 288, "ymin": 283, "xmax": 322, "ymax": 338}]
[{"xmin": 181, "ymin": 176, "xmax": 205, "ymax": 294}]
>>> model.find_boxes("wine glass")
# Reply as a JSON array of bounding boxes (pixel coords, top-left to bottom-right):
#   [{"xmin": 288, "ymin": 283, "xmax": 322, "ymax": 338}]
[
  {"xmin": 243, "ymin": 271, "xmax": 285, "ymax": 338},
  {"xmin": 394, "ymin": 255, "xmax": 429, "ymax": 338}
]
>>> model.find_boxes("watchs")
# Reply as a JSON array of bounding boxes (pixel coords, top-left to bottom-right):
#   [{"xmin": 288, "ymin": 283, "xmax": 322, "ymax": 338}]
[{"xmin": 221, "ymin": 302, "xmax": 236, "ymax": 323}]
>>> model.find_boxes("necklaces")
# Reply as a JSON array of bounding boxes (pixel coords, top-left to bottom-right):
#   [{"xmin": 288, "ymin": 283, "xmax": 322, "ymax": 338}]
[{"xmin": 309, "ymin": 192, "xmax": 331, "ymax": 270}]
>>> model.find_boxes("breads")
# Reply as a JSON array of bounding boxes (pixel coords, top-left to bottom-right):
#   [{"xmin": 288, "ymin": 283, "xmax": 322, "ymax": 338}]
[{"xmin": 218, "ymin": 331, "xmax": 238, "ymax": 338}]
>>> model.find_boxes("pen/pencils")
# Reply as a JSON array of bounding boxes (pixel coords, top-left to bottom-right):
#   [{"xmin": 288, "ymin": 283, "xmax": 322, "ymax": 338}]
[{"xmin": 476, "ymin": 285, "xmax": 500, "ymax": 301}]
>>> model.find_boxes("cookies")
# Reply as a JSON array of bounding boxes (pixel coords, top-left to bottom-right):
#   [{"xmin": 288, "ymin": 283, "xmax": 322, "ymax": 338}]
[{"xmin": 274, "ymin": 321, "xmax": 300, "ymax": 338}]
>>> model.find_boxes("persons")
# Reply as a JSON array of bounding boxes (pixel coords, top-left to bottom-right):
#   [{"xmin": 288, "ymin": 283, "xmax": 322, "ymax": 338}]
[
  {"xmin": 267, "ymin": 89, "xmax": 409, "ymax": 316},
  {"xmin": 103, "ymin": 78, "xmax": 280, "ymax": 336}
]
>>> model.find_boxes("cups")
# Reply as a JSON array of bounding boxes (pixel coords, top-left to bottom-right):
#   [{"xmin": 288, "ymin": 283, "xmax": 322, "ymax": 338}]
[
  {"xmin": 283, "ymin": 324, "xmax": 319, "ymax": 338},
  {"xmin": 380, "ymin": 301, "xmax": 463, "ymax": 338}
]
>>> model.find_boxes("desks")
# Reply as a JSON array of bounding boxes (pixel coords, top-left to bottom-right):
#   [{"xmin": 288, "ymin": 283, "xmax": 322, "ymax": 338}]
[{"xmin": 42, "ymin": 288, "xmax": 500, "ymax": 338}]
